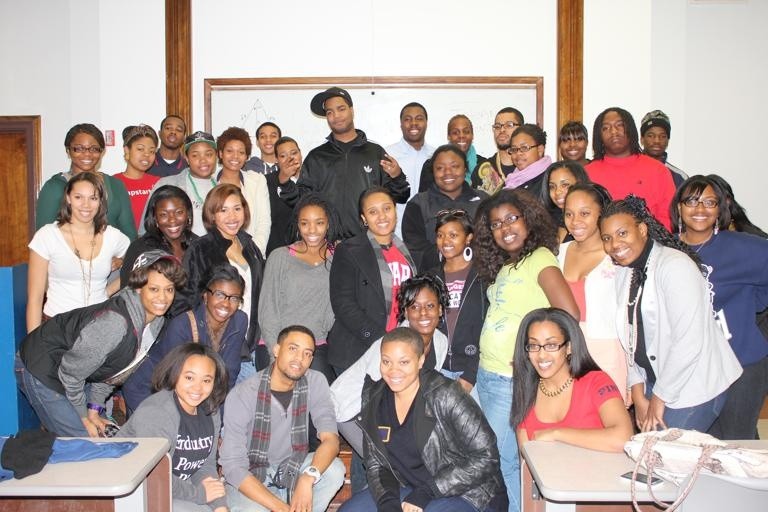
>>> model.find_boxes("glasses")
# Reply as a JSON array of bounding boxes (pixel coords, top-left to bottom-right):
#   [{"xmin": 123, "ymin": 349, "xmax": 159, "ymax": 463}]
[
  {"xmin": 522, "ymin": 341, "xmax": 568, "ymax": 353},
  {"xmin": 489, "ymin": 214, "xmax": 525, "ymax": 231},
  {"xmin": 682, "ymin": 197, "xmax": 719, "ymax": 210},
  {"xmin": 507, "ymin": 144, "xmax": 541, "ymax": 155},
  {"xmin": 68, "ymin": 144, "xmax": 102, "ymax": 153},
  {"xmin": 492, "ymin": 121, "xmax": 521, "ymax": 129}
]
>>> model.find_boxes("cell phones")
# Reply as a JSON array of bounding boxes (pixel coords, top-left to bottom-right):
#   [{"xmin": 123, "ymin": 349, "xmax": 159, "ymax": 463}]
[{"xmin": 622, "ymin": 470, "xmax": 663, "ymax": 486}]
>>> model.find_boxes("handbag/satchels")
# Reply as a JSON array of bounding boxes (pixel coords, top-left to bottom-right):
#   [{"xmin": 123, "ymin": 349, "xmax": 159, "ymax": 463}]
[{"xmin": 625, "ymin": 427, "xmax": 768, "ymax": 512}]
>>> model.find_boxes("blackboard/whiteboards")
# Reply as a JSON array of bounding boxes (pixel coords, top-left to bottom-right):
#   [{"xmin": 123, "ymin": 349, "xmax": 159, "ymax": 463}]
[{"xmin": 204, "ymin": 77, "xmax": 544, "ymax": 166}]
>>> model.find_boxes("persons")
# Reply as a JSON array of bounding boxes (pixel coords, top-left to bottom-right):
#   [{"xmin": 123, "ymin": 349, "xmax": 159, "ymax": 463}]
[
  {"xmin": 15, "ymin": 115, "xmax": 347, "ymax": 511},
  {"xmin": 324, "ymin": 187, "xmax": 511, "ymax": 511},
  {"xmin": 384, "ymin": 102, "xmax": 767, "ymax": 511},
  {"xmin": 276, "ymin": 88, "xmax": 411, "ymax": 243}
]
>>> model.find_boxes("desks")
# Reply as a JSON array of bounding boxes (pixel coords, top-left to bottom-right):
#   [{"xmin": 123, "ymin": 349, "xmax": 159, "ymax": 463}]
[
  {"xmin": 520, "ymin": 438, "xmax": 768, "ymax": 511},
  {"xmin": 0, "ymin": 434, "xmax": 174, "ymax": 512}
]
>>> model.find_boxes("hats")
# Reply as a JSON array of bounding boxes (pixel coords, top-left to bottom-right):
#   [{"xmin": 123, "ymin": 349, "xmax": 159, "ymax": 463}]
[
  {"xmin": 309, "ymin": 87, "xmax": 352, "ymax": 115},
  {"xmin": 183, "ymin": 130, "xmax": 216, "ymax": 149},
  {"xmin": 131, "ymin": 249, "xmax": 181, "ymax": 270},
  {"xmin": 641, "ymin": 110, "xmax": 672, "ymax": 132}
]
